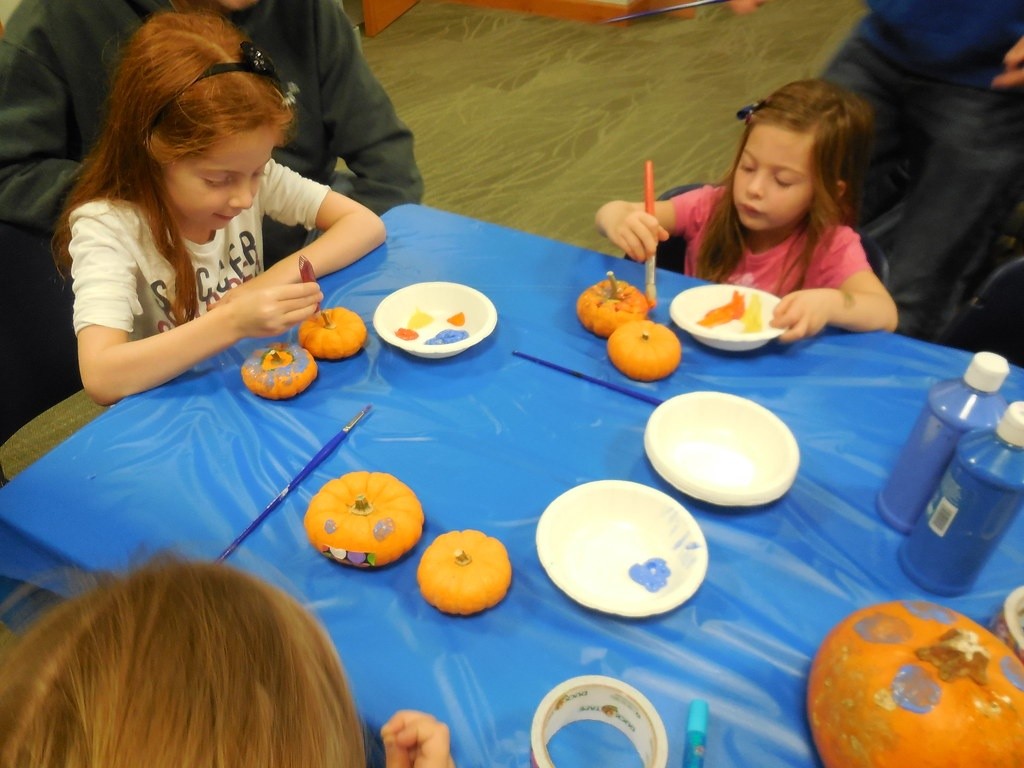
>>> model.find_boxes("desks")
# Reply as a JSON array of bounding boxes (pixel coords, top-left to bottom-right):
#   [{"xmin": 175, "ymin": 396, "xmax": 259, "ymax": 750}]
[{"xmin": 0, "ymin": 202, "xmax": 1024, "ymax": 767}]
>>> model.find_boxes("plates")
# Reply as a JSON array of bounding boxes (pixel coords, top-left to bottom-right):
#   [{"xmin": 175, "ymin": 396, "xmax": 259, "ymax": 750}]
[
  {"xmin": 671, "ymin": 283, "xmax": 788, "ymax": 351},
  {"xmin": 535, "ymin": 479, "xmax": 709, "ymax": 616},
  {"xmin": 644, "ymin": 389, "xmax": 801, "ymax": 507},
  {"xmin": 372, "ymin": 282, "xmax": 498, "ymax": 359}
]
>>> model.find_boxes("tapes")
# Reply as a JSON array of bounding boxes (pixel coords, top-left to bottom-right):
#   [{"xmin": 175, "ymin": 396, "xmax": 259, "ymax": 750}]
[{"xmin": 528, "ymin": 675, "xmax": 669, "ymax": 768}]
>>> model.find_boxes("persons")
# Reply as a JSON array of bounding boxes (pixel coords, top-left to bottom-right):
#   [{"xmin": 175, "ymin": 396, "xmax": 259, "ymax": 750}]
[
  {"xmin": 2, "ymin": 0, "xmax": 425, "ymax": 432},
  {"xmin": 818, "ymin": 0, "xmax": 1023, "ymax": 336},
  {"xmin": 2, "ymin": 552, "xmax": 454, "ymax": 766},
  {"xmin": 47, "ymin": 8, "xmax": 390, "ymax": 411},
  {"xmin": 592, "ymin": 74, "xmax": 901, "ymax": 345}
]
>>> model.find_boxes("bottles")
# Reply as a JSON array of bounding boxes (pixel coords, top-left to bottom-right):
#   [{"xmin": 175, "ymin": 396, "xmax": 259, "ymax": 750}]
[
  {"xmin": 877, "ymin": 349, "xmax": 1010, "ymax": 536},
  {"xmin": 895, "ymin": 401, "xmax": 1024, "ymax": 597}
]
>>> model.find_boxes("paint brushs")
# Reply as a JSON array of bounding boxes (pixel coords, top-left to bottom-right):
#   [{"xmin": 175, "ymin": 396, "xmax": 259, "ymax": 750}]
[
  {"xmin": 204, "ymin": 402, "xmax": 373, "ymax": 570},
  {"xmin": 512, "ymin": 349, "xmax": 663, "ymax": 405},
  {"xmin": 643, "ymin": 160, "xmax": 658, "ymax": 310}
]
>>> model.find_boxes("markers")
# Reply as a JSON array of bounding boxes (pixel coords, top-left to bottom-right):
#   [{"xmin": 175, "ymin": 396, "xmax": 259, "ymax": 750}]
[{"xmin": 682, "ymin": 699, "xmax": 709, "ymax": 768}]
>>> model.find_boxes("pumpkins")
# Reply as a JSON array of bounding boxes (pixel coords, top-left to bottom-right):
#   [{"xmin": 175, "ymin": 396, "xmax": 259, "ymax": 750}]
[
  {"xmin": 298, "ymin": 468, "xmax": 512, "ymax": 620},
  {"xmin": 808, "ymin": 599, "xmax": 1024, "ymax": 767},
  {"xmin": 240, "ymin": 302, "xmax": 367, "ymax": 399},
  {"xmin": 574, "ymin": 270, "xmax": 682, "ymax": 381}
]
